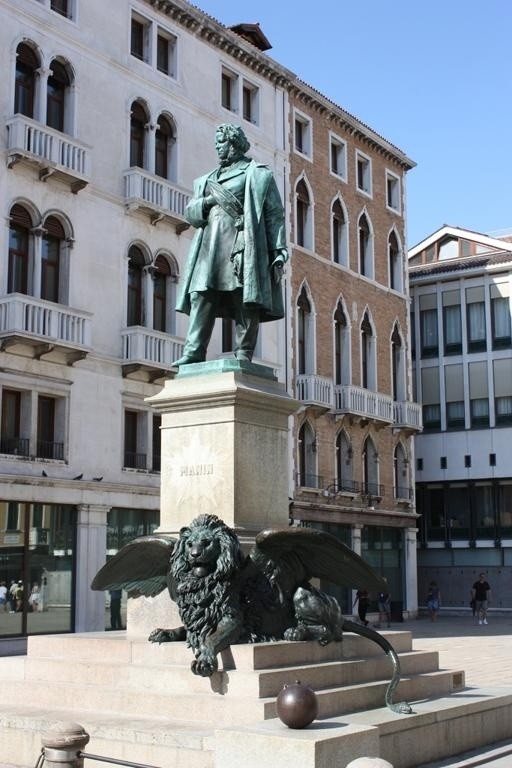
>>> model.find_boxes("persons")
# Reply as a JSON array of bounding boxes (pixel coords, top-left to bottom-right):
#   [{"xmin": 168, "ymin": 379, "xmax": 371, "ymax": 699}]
[
  {"xmin": 426, "ymin": 582, "xmax": 441, "ymax": 624},
  {"xmin": 1, "ymin": 580, "xmax": 41, "ymax": 615},
  {"xmin": 352, "ymin": 589, "xmax": 369, "ymax": 627},
  {"xmin": 471, "ymin": 572, "xmax": 492, "ymax": 625},
  {"xmin": 373, "ymin": 576, "xmax": 392, "ymax": 630},
  {"xmin": 109, "ymin": 589, "xmax": 123, "ymax": 629},
  {"xmin": 170, "ymin": 123, "xmax": 289, "ymax": 368}
]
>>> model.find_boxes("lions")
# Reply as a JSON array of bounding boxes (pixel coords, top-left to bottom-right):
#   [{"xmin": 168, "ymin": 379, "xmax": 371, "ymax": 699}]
[{"xmin": 147, "ymin": 513, "xmax": 413, "ymax": 716}]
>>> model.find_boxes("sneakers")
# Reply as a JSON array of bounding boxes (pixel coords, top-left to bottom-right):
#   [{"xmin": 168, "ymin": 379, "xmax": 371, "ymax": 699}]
[{"xmin": 478, "ymin": 618, "xmax": 488, "ymax": 625}]
